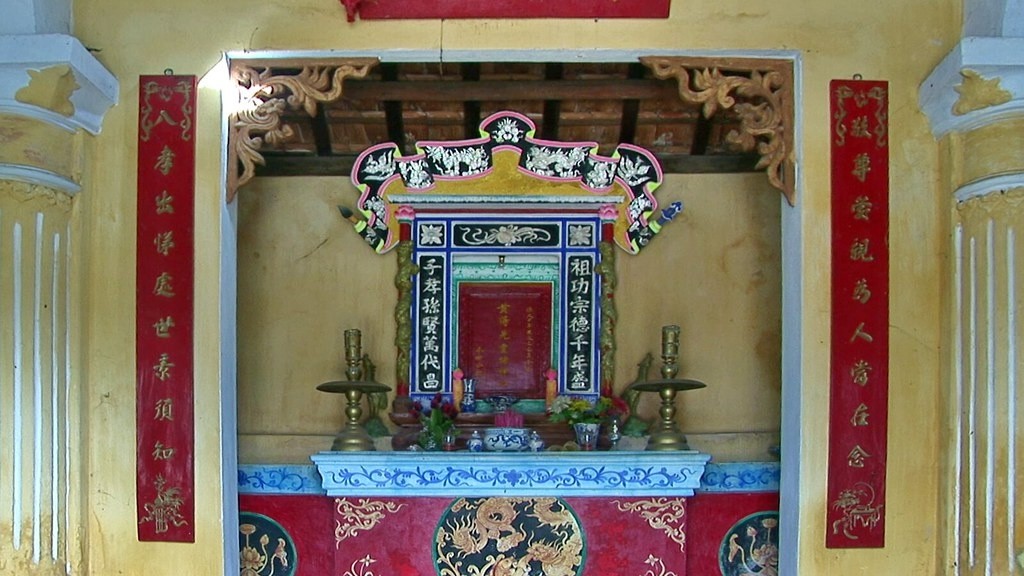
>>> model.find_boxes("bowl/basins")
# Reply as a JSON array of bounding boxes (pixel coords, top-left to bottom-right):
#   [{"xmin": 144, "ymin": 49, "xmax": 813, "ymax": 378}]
[{"xmin": 475, "ymin": 395, "xmax": 545, "ymax": 413}]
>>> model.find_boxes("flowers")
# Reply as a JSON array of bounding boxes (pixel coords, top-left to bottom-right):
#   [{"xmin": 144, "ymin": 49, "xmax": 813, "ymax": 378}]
[
  {"xmin": 544, "ymin": 384, "xmax": 629, "ymax": 428},
  {"xmin": 412, "ymin": 392, "xmax": 457, "ymax": 443}
]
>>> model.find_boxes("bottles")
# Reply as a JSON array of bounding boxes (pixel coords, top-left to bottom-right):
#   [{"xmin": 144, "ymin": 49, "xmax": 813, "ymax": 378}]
[
  {"xmin": 467, "ymin": 431, "xmax": 483, "ymax": 453},
  {"xmin": 530, "ymin": 431, "xmax": 546, "ymax": 452},
  {"xmin": 459, "ymin": 378, "xmax": 479, "ymax": 412}
]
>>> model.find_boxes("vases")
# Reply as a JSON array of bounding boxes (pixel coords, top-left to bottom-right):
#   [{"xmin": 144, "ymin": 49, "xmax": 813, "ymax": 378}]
[
  {"xmin": 421, "ymin": 425, "xmax": 440, "ymax": 450},
  {"xmin": 572, "ymin": 423, "xmax": 600, "ymax": 452}
]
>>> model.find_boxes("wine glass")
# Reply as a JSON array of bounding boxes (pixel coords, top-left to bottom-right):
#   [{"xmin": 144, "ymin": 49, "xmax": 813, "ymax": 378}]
[{"xmin": 608, "ymin": 420, "xmax": 621, "ymax": 451}]
